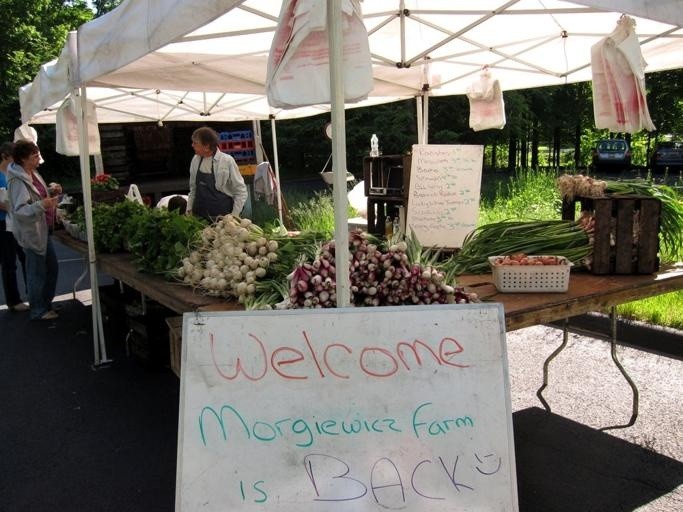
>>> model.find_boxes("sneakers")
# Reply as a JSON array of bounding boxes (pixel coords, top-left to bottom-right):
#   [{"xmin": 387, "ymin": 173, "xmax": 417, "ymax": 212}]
[{"xmin": 11, "ymin": 304, "xmax": 63, "ymax": 321}]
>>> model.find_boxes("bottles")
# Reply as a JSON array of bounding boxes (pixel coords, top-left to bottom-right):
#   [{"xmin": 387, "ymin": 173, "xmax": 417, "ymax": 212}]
[{"xmin": 369, "ymin": 134, "xmax": 379, "ymax": 158}]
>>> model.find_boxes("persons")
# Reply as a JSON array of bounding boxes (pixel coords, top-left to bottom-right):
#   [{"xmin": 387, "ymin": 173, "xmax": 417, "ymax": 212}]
[
  {"xmin": 0, "ymin": 141, "xmax": 62, "ymax": 319},
  {"xmin": 186, "ymin": 126, "xmax": 249, "ymax": 217},
  {"xmin": 156, "ymin": 194, "xmax": 190, "ymax": 214}
]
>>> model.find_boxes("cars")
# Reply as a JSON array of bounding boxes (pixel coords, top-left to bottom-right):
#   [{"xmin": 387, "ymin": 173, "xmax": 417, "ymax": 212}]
[
  {"xmin": 648, "ymin": 140, "xmax": 683, "ymax": 172},
  {"xmin": 590, "ymin": 137, "xmax": 633, "ymax": 169}
]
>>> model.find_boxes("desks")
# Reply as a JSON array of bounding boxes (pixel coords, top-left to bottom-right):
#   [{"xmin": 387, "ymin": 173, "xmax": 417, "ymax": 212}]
[{"xmin": 45, "ymin": 225, "xmax": 683, "ymax": 441}]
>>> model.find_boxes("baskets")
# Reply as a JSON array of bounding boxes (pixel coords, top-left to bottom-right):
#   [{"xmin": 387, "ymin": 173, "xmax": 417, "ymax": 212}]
[{"xmin": 486, "ymin": 254, "xmax": 574, "ymax": 294}]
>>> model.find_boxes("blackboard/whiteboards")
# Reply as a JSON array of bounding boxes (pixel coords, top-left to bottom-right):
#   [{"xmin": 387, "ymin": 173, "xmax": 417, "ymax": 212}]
[
  {"xmin": 175, "ymin": 303, "xmax": 519, "ymax": 512},
  {"xmin": 406, "ymin": 144, "xmax": 486, "ymax": 249}
]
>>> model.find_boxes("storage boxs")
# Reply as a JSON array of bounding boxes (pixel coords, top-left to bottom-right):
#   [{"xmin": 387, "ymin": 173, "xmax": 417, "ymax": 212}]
[{"xmin": 363, "ymin": 155, "xmax": 411, "ymax": 240}]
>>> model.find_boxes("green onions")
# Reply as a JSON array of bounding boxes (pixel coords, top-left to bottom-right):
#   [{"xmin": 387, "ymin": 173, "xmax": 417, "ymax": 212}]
[{"xmin": 459, "ymin": 175, "xmax": 683, "ymax": 274}]
